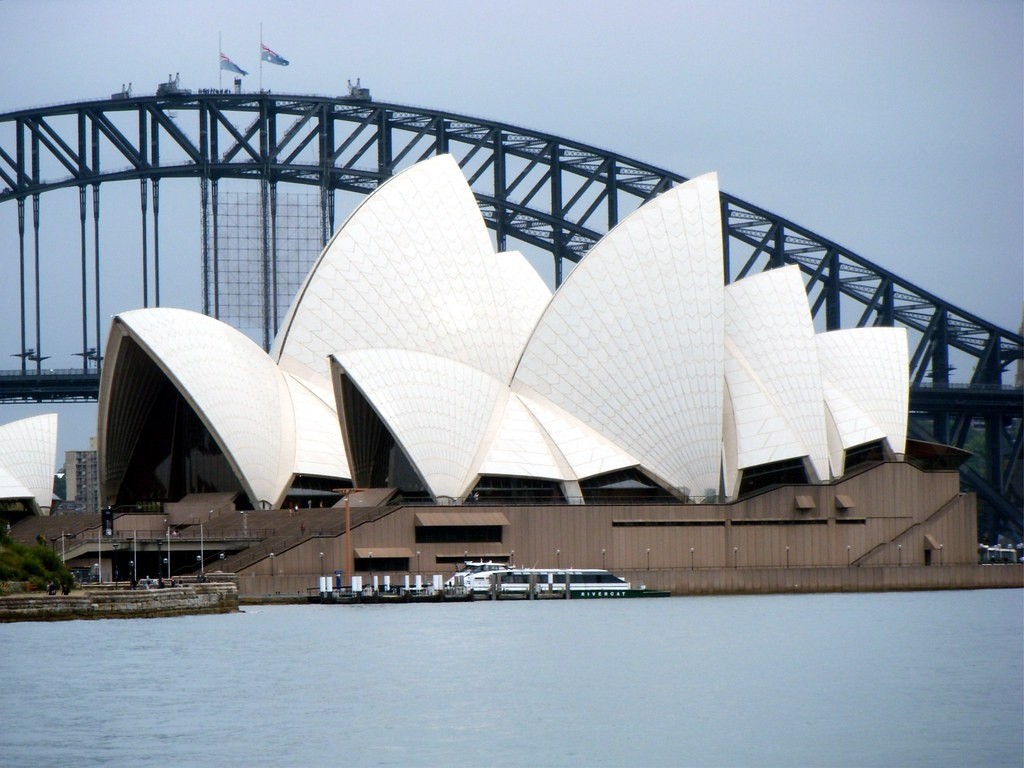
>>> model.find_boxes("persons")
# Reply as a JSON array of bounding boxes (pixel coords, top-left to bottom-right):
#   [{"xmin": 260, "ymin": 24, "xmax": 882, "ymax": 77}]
[
  {"xmin": 466, "ymin": 492, "xmax": 474, "ymax": 504},
  {"xmin": 169, "ymin": 576, "xmax": 175, "ymax": 587},
  {"xmin": 293, "ymin": 503, "xmax": 299, "ymax": 515},
  {"xmin": 128, "ymin": 571, "xmax": 136, "ymax": 590},
  {"xmin": 178, "ymin": 576, "xmax": 183, "ymax": 587},
  {"xmin": 474, "ymin": 491, "xmax": 480, "ymax": 504},
  {"xmin": 6, "ymin": 521, "xmax": 11, "ymax": 530},
  {"xmin": 993, "ymin": 543, "xmax": 1024, "ymax": 564},
  {"xmin": 335, "ymin": 573, "xmax": 347, "ymax": 593},
  {"xmin": 61, "ymin": 583, "xmax": 70, "ymax": 596},
  {"xmin": 144, "ymin": 578, "xmax": 155, "ymax": 589},
  {"xmin": 308, "ymin": 500, "xmax": 312, "ymax": 509},
  {"xmin": 197, "ymin": 572, "xmax": 206, "ymax": 583},
  {"xmin": 289, "ymin": 507, "xmax": 292, "ymax": 516},
  {"xmin": 156, "ymin": 572, "xmax": 166, "ymax": 589},
  {"xmin": 46, "ymin": 580, "xmax": 56, "ymax": 595}
]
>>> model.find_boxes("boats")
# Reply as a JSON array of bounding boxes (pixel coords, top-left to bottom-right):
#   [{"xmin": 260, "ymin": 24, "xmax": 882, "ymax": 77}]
[
  {"xmin": 444, "ymin": 559, "xmax": 510, "ymax": 586},
  {"xmin": 464, "ymin": 569, "xmax": 671, "ymax": 597}
]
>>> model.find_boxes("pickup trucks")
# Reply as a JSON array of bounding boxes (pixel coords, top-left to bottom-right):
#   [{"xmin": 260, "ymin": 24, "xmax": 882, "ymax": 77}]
[{"xmin": 130, "ymin": 575, "xmax": 159, "ymax": 589}]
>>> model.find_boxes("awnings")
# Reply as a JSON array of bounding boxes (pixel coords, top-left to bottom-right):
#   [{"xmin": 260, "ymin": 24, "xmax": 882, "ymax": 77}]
[
  {"xmin": 353, "ymin": 548, "xmax": 415, "ymax": 557},
  {"xmin": 414, "ymin": 512, "xmax": 511, "ymax": 526},
  {"xmin": 922, "ymin": 531, "xmax": 942, "ymax": 550},
  {"xmin": 437, "ymin": 553, "xmax": 513, "ymax": 558},
  {"xmin": 836, "ymin": 493, "xmax": 855, "ymax": 510},
  {"xmin": 796, "ymin": 495, "xmax": 816, "ymax": 511}
]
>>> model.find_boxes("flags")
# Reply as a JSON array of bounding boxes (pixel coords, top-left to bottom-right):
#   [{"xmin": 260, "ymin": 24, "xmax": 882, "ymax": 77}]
[
  {"xmin": 262, "ymin": 43, "xmax": 288, "ymax": 66},
  {"xmin": 220, "ymin": 50, "xmax": 248, "ymax": 76}
]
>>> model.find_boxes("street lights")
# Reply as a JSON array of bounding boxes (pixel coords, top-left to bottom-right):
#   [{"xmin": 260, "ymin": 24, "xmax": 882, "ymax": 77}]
[
  {"xmin": 163, "ymin": 558, "xmax": 168, "ymax": 578},
  {"xmin": 690, "ymin": 548, "xmax": 695, "ymax": 571},
  {"xmin": 94, "ymin": 563, "xmax": 98, "ymax": 582},
  {"xmin": 62, "ymin": 534, "xmax": 73, "ymax": 567},
  {"xmin": 940, "ymin": 544, "xmax": 943, "ymax": 567},
  {"xmin": 240, "ymin": 511, "xmax": 244, "ymax": 538},
  {"xmin": 786, "ymin": 547, "xmax": 790, "ymax": 568},
  {"xmin": 197, "ymin": 556, "xmax": 201, "ymax": 572},
  {"xmin": 464, "ymin": 551, "xmax": 468, "ymax": 561},
  {"xmin": 320, "ymin": 552, "xmax": 324, "ymax": 576},
  {"xmin": 647, "ymin": 548, "xmax": 650, "ymax": 570},
  {"xmin": 164, "ymin": 519, "xmax": 167, "ymax": 539},
  {"xmin": 898, "ymin": 544, "xmax": 901, "ymax": 566},
  {"xmin": 734, "ymin": 547, "xmax": 737, "ymax": 568},
  {"xmin": 209, "ymin": 509, "xmax": 213, "ymax": 521},
  {"xmin": 129, "ymin": 560, "xmax": 134, "ymax": 582},
  {"xmin": 557, "ymin": 549, "xmax": 560, "ymax": 569},
  {"xmin": 369, "ymin": 552, "xmax": 372, "ymax": 576},
  {"xmin": 270, "ymin": 553, "xmax": 274, "ymax": 576},
  {"xmin": 511, "ymin": 550, "xmax": 514, "ymax": 566},
  {"xmin": 417, "ymin": 551, "xmax": 420, "ymax": 574},
  {"xmin": 602, "ymin": 549, "xmax": 605, "ymax": 570},
  {"xmin": 847, "ymin": 545, "xmax": 850, "ymax": 567},
  {"xmin": 220, "ymin": 554, "xmax": 224, "ymax": 571},
  {"xmin": 51, "ymin": 538, "xmax": 57, "ymax": 558}
]
